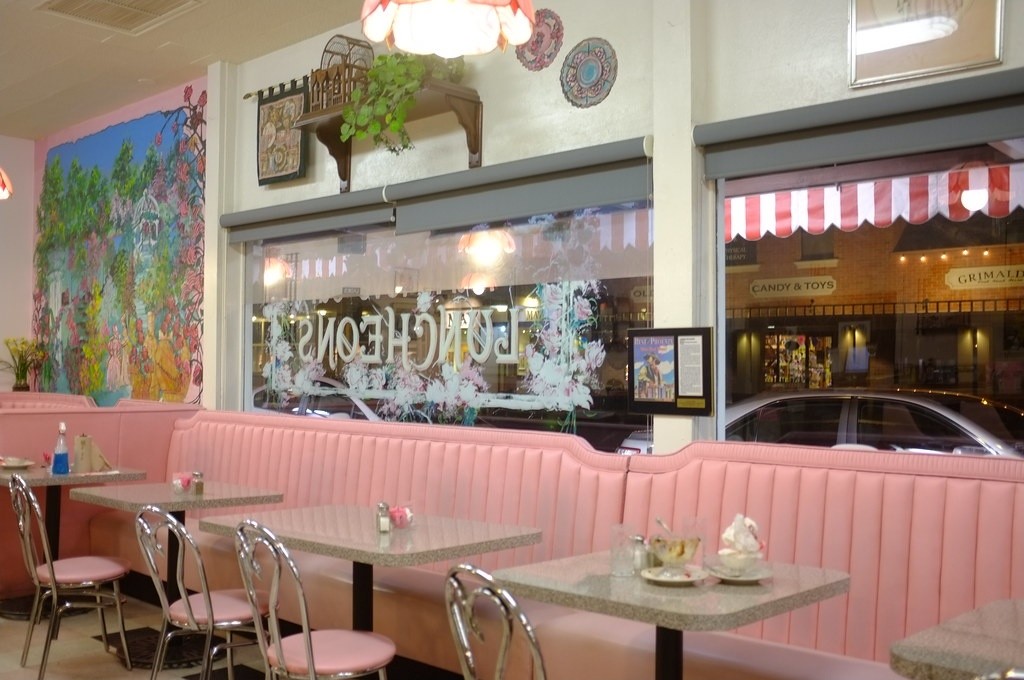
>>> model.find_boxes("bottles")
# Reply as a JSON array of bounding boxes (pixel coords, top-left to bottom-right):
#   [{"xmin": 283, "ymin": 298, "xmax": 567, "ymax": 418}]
[
  {"xmin": 190, "ymin": 471, "xmax": 204, "ymax": 496},
  {"xmin": 376, "ymin": 502, "xmax": 391, "ymax": 533},
  {"xmin": 634, "ymin": 536, "xmax": 647, "ymax": 570}
]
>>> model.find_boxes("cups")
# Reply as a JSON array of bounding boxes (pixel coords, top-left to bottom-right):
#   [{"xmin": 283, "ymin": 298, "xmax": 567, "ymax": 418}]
[{"xmin": 608, "ymin": 525, "xmax": 636, "ymax": 577}]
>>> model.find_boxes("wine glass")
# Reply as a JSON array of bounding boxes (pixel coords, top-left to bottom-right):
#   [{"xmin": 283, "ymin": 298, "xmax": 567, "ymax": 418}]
[{"xmin": 650, "ymin": 535, "xmax": 700, "ymax": 577}]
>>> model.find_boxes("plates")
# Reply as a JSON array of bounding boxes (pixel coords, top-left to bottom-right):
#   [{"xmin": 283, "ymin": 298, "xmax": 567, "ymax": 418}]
[
  {"xmin": 712, "ymin": 568, "xmax": 771, "ymax": 583},
  {"xmin": 0, "ymin": 458, "xmax": 35, "ymax": 469},
  {"xmin": 641, "ymin": 565, "xmax": 711, "ymax": 584}
]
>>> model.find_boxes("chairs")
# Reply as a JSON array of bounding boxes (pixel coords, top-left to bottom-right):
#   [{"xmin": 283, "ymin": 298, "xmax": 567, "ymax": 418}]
[
  {"xmin": 807, "ymin": 401, "xmax": 840, "ymax": 445},
  {"xmin": 232, "ymin": 517, "xmax": 396, "ymax": 680},
  {"xmin": 135, "ymin": 502, "xmax": 280, "ymax": 680},
  {"xmin": 7, "ymin": 470, "xmax": 134, "ymax": 680},
  {"xmin": 443, "ymin": 563, "xmax": 547, "ymax": 680}
]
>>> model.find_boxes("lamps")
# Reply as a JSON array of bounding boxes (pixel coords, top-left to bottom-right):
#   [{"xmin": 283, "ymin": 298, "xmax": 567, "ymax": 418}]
[{"xmin": 359, "ymin": 0, "xmax": 537, "ymax": 60}]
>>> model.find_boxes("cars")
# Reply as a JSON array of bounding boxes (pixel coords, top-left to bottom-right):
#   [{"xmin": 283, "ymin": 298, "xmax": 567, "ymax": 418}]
[
  {"xmin": 614, "ymin": 384, "xmax": 1024, "ymax": 471},
  {"xmin": 252, "ymin": 375, "xmax": 434, "ymax": 425}
]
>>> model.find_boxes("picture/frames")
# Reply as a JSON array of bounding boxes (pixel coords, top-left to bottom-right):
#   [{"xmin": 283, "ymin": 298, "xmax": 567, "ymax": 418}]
[{"xmin": 845, "ymin": 0, "xmax": 1005, "ymax": 89}]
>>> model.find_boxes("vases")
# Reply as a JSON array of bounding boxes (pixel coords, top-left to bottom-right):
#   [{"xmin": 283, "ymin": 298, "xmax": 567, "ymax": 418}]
[{"xmin": 12, "ymin": 367, "xmax": 30, "ymax": 391}]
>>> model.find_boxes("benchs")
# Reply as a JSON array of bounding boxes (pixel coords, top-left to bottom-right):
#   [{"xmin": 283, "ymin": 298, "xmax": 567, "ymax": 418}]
[{"xmin": 1, "ymin": 390, "xmax": 1024, "ymax": 680}]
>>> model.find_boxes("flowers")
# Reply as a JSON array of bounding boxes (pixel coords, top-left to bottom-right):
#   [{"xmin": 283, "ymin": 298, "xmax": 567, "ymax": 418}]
[{"xmin": 0, "ymin": 336, "xmax": 44, "ymax": 386}]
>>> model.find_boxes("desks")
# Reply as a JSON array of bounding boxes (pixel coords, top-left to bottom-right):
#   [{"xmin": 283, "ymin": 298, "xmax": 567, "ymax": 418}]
[
  {"xmin": 68, "ymin": 480, "xmax": 284, "ymax": 670},
  {"xmin": 1, "ymin": 464, "xmax": 147, "ymax": 623},
  {"xmin": 491, "ymin": 546, "xmax": 853, "ymax": 680},
  {"xmin": 200, "ymin": 501, "xmax": 543, "ymax": 680},
  {"xmin": 888, "ymin": 597, "xmax": 1024, "ymax": 680}
]
